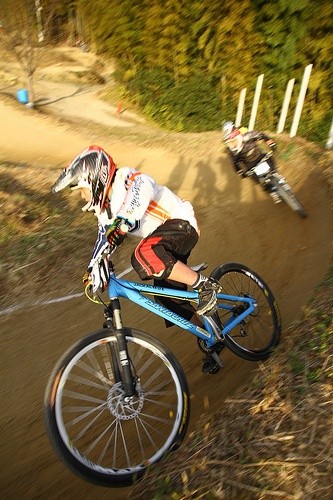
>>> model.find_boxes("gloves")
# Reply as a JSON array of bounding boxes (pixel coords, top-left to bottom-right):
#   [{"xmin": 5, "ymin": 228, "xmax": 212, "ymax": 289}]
[
  {"xmin": 268, "ymin": 140, "xmax": 278, "ymax": 155},
  {"xmin": 91, "ymin": 246, "xmax": 113, "ymax": 292},
  {"xmin": 237, "ymin": 170, "xmax": 246, "ymax": 181}
]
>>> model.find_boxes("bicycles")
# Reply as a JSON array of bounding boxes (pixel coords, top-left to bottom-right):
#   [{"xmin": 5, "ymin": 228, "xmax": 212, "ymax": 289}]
[
  {"xmin": 42, "ymin": 225, "xmax": 281, "ymax": 488},
  {"xmin": 238, "ymin": 142, "xmax": 308, "ymax": 218}
]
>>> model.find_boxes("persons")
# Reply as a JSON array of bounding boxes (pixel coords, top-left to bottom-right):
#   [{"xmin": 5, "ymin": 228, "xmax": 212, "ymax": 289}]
[
  {"xmin": 51, "ymin": 146, "xmax": 228, "ymax": 374},
  {"xmin": 222, "ymin": 123, "xmax": 290, "ymax": 206}
]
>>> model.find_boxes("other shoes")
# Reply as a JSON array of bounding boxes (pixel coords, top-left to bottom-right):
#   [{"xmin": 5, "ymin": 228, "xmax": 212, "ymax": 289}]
[
  {"xmin": 271, "ymin": 190, "xmax": 283, "ymax": 205},
  {"xmin": 280, "ymin": 179, "xmax": 292, "ymax": 190}
]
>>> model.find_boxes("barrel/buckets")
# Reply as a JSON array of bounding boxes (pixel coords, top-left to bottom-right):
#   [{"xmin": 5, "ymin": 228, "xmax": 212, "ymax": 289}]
[{"xmin": 17, "ymin": 90, "xmax": 28, "ymax": 103}]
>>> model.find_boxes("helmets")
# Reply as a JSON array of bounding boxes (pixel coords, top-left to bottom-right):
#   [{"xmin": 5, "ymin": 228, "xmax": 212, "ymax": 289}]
[
  {"xmin": 50, "ymin": 144, "xmax": 117, "ymax": 218},
  {"xmin": 221, "ymin": 123, "xmax": 243, "ymax": 153}
]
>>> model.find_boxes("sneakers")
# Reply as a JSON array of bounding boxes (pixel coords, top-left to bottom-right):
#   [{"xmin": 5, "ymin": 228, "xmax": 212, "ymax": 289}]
[
  {"xmin": 200, "ymin": 341, "xmax": 227, "ymax": 374},
  {"xmin": 194, "ymin": 277, "xmax": 223, "ymax": 316}
]
